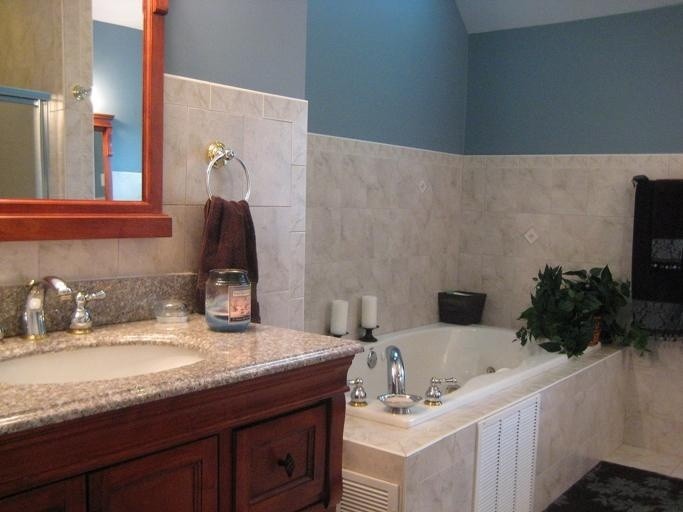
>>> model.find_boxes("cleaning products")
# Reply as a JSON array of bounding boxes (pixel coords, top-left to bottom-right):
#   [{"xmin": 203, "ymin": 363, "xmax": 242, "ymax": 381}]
[{"xmin": 150, "ymin": 299, "xmax": 192, "ymax": 324}]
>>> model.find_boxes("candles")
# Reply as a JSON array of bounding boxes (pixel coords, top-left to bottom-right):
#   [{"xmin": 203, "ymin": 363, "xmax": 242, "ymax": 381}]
[
  {"xmin": 329, "ymin": 300, "xmax": 348, "ymax": 335},
  {"xmin": 361, "ymin": 295, "xmax": 378, "ymax": 329}
]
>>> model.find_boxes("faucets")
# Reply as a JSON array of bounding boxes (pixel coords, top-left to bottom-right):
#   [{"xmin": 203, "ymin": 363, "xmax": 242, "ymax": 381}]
[
  {"xmin": 385, "ymin": 345, "xmax": 412, "ymax": 415},
  {"xmin": 24, "ymin": 275, "xmax": 70, "ymax": 340}
]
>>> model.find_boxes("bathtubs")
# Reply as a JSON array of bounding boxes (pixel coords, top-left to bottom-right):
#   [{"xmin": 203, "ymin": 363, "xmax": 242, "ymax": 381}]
[{"xmin": 336, "ymin": 324, "xmax": 629, "ymax": 512}]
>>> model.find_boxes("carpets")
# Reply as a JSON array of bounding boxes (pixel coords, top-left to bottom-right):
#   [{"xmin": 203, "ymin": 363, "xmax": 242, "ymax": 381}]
[{"xmin": 541, "ymin": 460, "xmax": 683, "ymax": 512}]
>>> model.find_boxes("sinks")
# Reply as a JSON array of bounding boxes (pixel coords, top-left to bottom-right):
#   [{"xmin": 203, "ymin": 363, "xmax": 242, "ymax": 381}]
[{"xmin": 0, "ymin": 340, "xmax": 205, "ymax": 386}]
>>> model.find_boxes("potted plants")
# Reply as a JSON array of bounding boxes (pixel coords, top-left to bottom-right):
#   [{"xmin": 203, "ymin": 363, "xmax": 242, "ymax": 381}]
[{"xmin": 511, "ymin": 262, "xmax": 652, "ymax": 360}]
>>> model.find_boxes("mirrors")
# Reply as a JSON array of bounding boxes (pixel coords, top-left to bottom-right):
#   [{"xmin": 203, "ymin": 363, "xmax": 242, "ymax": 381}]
[
  {"xmin": 94, "ymin": 113, "xmax": 115, "ymax": 201},
  {"xmin": 0, "ymin": 0, "xmax": 173, "ymax": 243}
]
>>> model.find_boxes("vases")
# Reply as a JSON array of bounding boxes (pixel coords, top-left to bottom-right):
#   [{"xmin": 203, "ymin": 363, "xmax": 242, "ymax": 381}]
[{"xmin": 587, "ymin": 315, "xmax": 603, "ymax": 346}]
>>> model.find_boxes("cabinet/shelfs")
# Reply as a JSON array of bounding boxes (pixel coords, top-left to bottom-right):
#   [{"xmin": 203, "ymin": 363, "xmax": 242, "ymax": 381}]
[
  {"xmin": 233, "ymin": 355, "xmax": 353, "ymax": 512},
  {"xmin": 84, "ymin": 384, "xmax": 233, "ymax": 512},
  {"xmin": 0, "ymin": 418, "xmax": 86, "ymax": 512}
]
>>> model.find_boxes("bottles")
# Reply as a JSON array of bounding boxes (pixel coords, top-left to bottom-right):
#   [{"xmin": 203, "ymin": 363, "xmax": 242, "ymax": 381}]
[{"xmin": 205, "ymin": 269, "xmax": 251, "ymax": 330}]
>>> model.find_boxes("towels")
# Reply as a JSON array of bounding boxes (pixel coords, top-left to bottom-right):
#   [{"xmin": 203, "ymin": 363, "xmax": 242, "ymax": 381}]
[{"xmin": 195, "ymin": 195, "xmax": 262, "ymax": 325}]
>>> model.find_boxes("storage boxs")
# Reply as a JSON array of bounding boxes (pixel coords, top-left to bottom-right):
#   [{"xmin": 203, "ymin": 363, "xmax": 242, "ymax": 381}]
[{"xmin": 438, "ymin": 291, "xmax": 486, "ymax": 325}]
